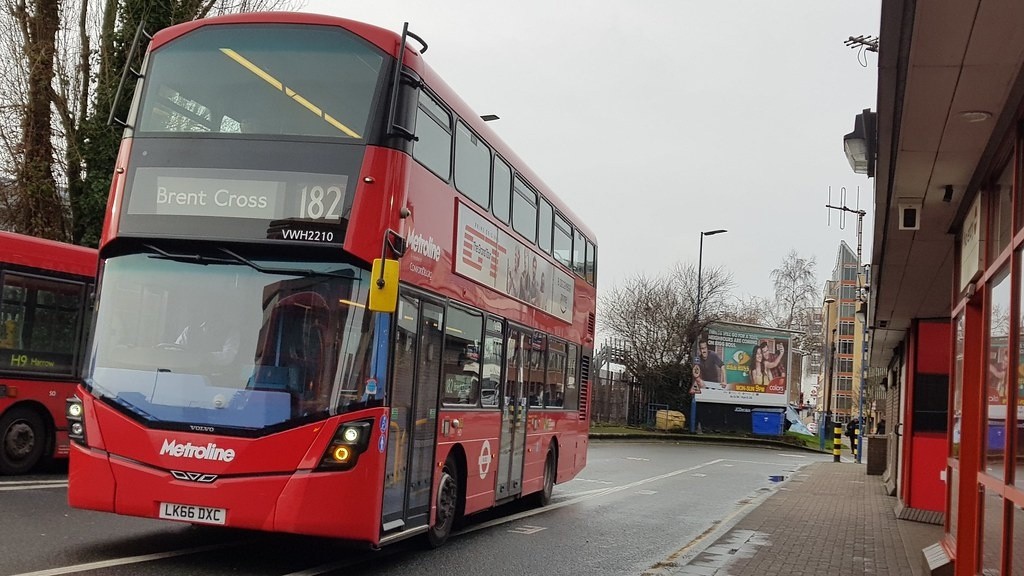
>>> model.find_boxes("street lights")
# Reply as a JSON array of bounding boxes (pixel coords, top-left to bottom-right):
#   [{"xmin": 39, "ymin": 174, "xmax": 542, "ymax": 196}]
[{"xmin": 687, "ymin": 228, "xmax": 729, "ymax": 431}]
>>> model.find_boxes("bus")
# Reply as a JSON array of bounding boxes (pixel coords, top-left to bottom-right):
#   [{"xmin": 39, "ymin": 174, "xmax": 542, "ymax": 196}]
[
  {"xmin": 1, "ymin": 227, "xmax": 105, "ymax": 477},
  {"xmin": 62, "ymin": 12, "xmax": 599, "ymax": 554}
]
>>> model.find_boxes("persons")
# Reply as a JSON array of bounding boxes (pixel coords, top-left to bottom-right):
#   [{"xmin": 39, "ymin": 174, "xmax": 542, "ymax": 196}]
[
  {"xmin": 505, "ymin": 246, "xmax": 519, "ymax": 297},
  {"xmin": 520, "ymin": 248, "xmax": 531, "ymax": 301},
  {"xmin": 984, "ymin": 344, "xmax": 1007, "ymax": 400},
  {"xmin": 875, "ymin": 416, "xmax": 886, "ymax": 433},
  {"xmin": 748, "ymin": 343, "xmax": 785, "ymax": 386},
  {"xmin": 528, "ymin": 256, "xmax": 539, "ymax": 306},
  {"xmin": 537, "ymin": 271, "xmax": 547, "ymax": 311},
  {"xmin": 162, "ymin": 291, "xmax": 242, "ymax": 365},
  {"xmin": 846, "ymin": 417, "xmax": 857, "ymax": 454},
  {"xmin": 456, "ymin": 385, "xmax": 562, "ymax": 421},
  {"xmin": 695, "ymin": 339, "xmax": 726, "ymax": 388}
]
